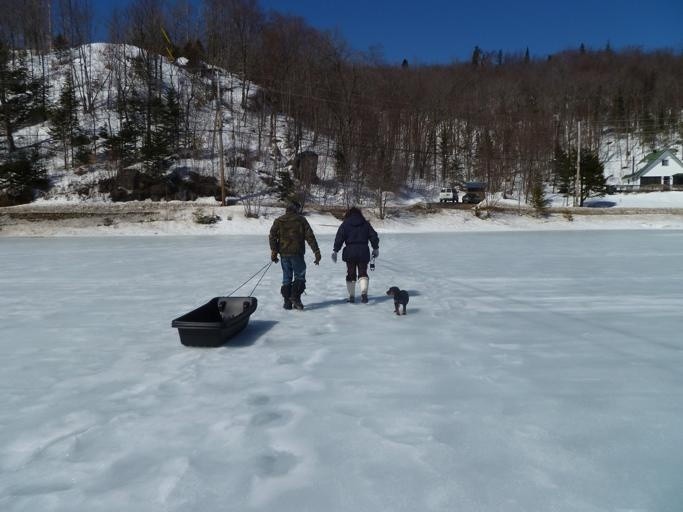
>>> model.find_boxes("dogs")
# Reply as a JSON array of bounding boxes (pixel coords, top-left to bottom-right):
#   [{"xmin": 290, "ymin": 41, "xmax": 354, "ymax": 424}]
[{"xmin": 386, "ymin": 286, "xmax": 410, "ymax": 316}]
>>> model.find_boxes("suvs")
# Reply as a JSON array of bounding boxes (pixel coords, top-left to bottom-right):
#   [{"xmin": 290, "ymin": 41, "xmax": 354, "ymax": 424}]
[{"xmin": 439, "ymin": 188, "xmax": 458, "ymax": 203}]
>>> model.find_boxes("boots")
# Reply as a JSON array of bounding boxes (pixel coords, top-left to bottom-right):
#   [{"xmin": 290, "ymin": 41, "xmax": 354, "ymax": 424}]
[
  {"xmin": 280, "ymin": 284, "xmax": 292, "ymax": 309},
  {"xmin": 357, "ymin": 277, "xmax": 369, "ymax": 303},
  {"xmin": 345, "ymin": 280, "xmax": 356, "ymax": 302},
  {"xmin": 289, "ymin": 280, "xmax": 306, "ymax": 310}
]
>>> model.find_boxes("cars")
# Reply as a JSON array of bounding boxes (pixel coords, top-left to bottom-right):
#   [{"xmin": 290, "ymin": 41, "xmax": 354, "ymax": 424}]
[{"xmin": 462, "ymin": 192, "xmax": 482, "ymax": 204}]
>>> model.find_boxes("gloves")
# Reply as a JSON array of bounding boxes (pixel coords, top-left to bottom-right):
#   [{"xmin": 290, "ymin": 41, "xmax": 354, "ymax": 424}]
[
  {"xmin": 331, "ymin": 251, "xmax": 337, "ymax": 263},
  {"xmin": 313, "ymin": 251, "xmax": 321, "ymax": 265},
  {"xmin": 271, "ymin": 252, "xmax": 279, "ymax": 263},
  {"xmin": 371, "ymin": 248, "xmax": 379, "ymax": 258}
]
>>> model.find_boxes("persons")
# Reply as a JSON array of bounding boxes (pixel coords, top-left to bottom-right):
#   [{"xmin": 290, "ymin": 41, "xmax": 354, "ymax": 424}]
[
  {"xmin": 330, "ymin": 206, "xmax": 379, "ymax": 304},
  {"xmin": 268, "ymin": 201, "xmax": 321, "ymax": 311}
]
